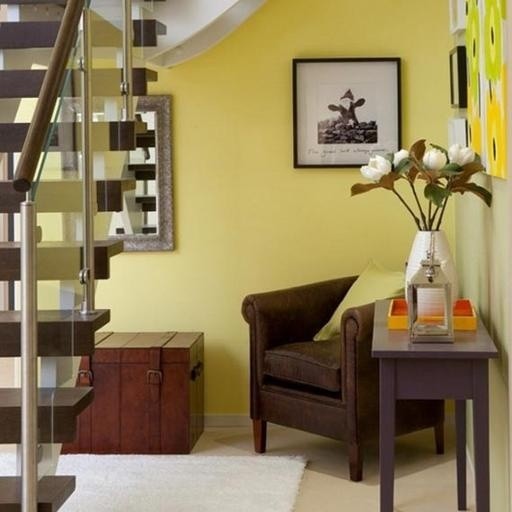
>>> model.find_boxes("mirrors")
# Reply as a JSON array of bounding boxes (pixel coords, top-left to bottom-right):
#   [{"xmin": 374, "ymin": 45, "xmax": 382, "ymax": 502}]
[{"xmin": 58, "ymin": 93, "xmax": 177, "ymax": 253}]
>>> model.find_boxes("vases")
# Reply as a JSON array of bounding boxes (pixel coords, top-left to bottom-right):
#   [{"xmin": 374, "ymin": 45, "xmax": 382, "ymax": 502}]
[{"xmin": 405, "ymin": 229, "xmax": 460, "ymax": 317}]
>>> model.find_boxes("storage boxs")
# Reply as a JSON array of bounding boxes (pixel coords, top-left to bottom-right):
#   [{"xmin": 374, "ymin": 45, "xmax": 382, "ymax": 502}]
[{"xmin": 58, "ymin": 332, "xmax": 207, "ymax": 457}]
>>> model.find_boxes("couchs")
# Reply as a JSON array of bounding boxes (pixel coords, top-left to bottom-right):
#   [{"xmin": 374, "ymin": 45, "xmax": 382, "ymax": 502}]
[{"xmin": 240, "ymin": 275, "xmax": 447, "ymax": 484}]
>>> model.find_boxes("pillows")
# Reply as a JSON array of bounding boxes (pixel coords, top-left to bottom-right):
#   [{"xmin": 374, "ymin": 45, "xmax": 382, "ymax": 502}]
[{"xmin": 311, "ymin": 259, "xmax": 406, "ymax": 343}]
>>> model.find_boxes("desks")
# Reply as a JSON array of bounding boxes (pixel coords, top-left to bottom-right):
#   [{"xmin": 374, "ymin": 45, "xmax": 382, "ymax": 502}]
[{"xmin": 371, "ymin": 298, "xmax": 498, "ymax": 512}]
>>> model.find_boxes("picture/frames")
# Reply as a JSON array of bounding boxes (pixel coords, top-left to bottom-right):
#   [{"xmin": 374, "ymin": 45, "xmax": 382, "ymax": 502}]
[
  {"xmin": 449, "ymin": 45, "xmax": 468, "ymax": 108},
  {"xmin": 448, "ymin": 0, "xmax": 466, "ymax": 36},
  {"xmin": 447, "ymin": 118, "xmax": 469, "ymax": 151},
  {"xmin": 405, "ymin": 281, "xmax": 455, "ymax": 343},
  {"xmin": 292, "ymin": 55, "xmax": 403, "ymax": 169}
]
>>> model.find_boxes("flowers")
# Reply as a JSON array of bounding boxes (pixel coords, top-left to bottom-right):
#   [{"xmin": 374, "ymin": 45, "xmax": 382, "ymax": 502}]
[{"xmin": 349, "ymin": 138, "xmax": 493, "ymax": 231}]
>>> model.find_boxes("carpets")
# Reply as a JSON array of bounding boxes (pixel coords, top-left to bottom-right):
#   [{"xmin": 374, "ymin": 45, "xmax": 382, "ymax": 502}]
[{"xmin": 1, "ymin": 448, "xmax": 312, "ymax": 512}]
